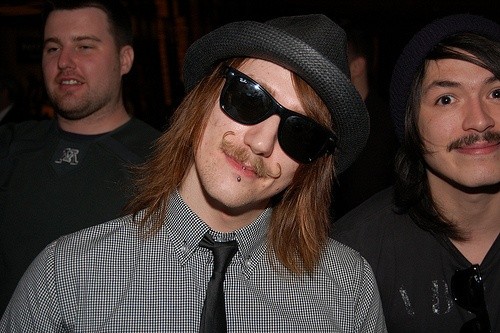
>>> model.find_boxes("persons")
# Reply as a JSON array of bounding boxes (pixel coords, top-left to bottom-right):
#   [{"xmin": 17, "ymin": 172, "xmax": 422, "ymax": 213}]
[
  {"xmin": 346, "ymin": 44, "xmax": 390, "ymax": 103},
  {"xmin": 330, "ymin": 13, "xmax": 500, "ymax": 333},
  {"xmin": 0, "ymin": 0, "xmax": 165, "ymax": 319},
  {"xmin": 0, "ymin": 13, "xmax": 388, "ymax": 333}
]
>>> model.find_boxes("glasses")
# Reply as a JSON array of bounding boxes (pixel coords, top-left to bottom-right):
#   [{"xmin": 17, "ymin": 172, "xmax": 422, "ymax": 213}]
[
  {"xmin": 218, "ymin": 64, "xmax": 336, "ymax": 167},
  {"xmin": 450, "ymin": 264, "xmax": 493, "ymax": 333}
]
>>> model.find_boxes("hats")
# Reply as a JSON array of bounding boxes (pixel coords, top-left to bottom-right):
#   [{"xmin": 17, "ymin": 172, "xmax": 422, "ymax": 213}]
[{"xmin": 183, "ymin": 13, "xmax": 371, "ymax": 177}]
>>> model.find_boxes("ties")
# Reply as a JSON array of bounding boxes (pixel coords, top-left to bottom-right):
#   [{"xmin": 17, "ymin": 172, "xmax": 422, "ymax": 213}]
[{"xmin": 198, "ymin": 232, "xmax": 239, "ymax": 333}]
